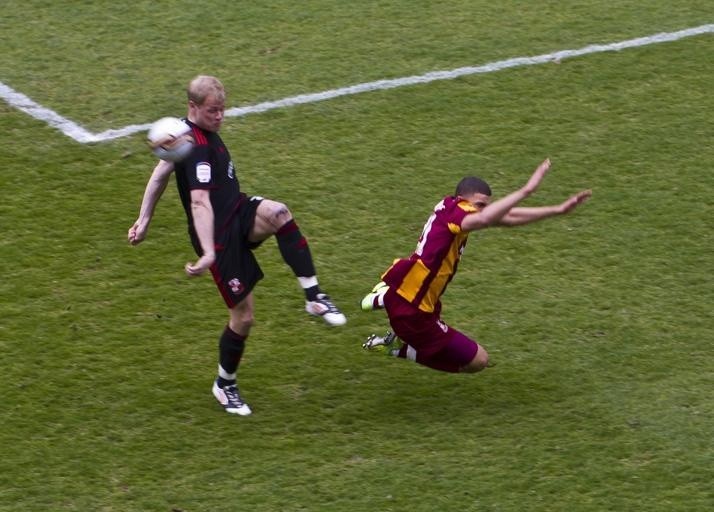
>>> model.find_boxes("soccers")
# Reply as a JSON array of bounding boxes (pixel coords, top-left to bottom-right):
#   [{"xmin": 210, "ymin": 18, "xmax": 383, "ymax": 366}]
[{"xmin": 148, "ymin": 114, "xmax": 197, "ymax": 166}]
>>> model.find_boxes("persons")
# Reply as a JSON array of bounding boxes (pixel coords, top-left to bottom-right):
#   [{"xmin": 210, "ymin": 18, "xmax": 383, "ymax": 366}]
[
  {"xmin": 125, "ymin": 74, "xmax": 347, "ymax": 414},
  {"xmin": 359, "ymin": 157, "xmax": 595, "ymax": 375}
]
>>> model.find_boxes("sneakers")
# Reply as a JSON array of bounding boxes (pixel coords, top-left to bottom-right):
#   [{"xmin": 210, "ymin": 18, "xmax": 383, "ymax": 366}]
[
  {"xmin": 212, "ymin": 379, "xmax": 253, "ymax": 417},
  {"xmin": 304, "ymin": 293, "xmax": 347, "ymax": 327},
  {"xmin": 363, "ymin": 331, "xmax": 401, "ymax": 357},
  {"xmin": 361, "ymin": 280, "xmax": 388, "ymax": 311}
]
